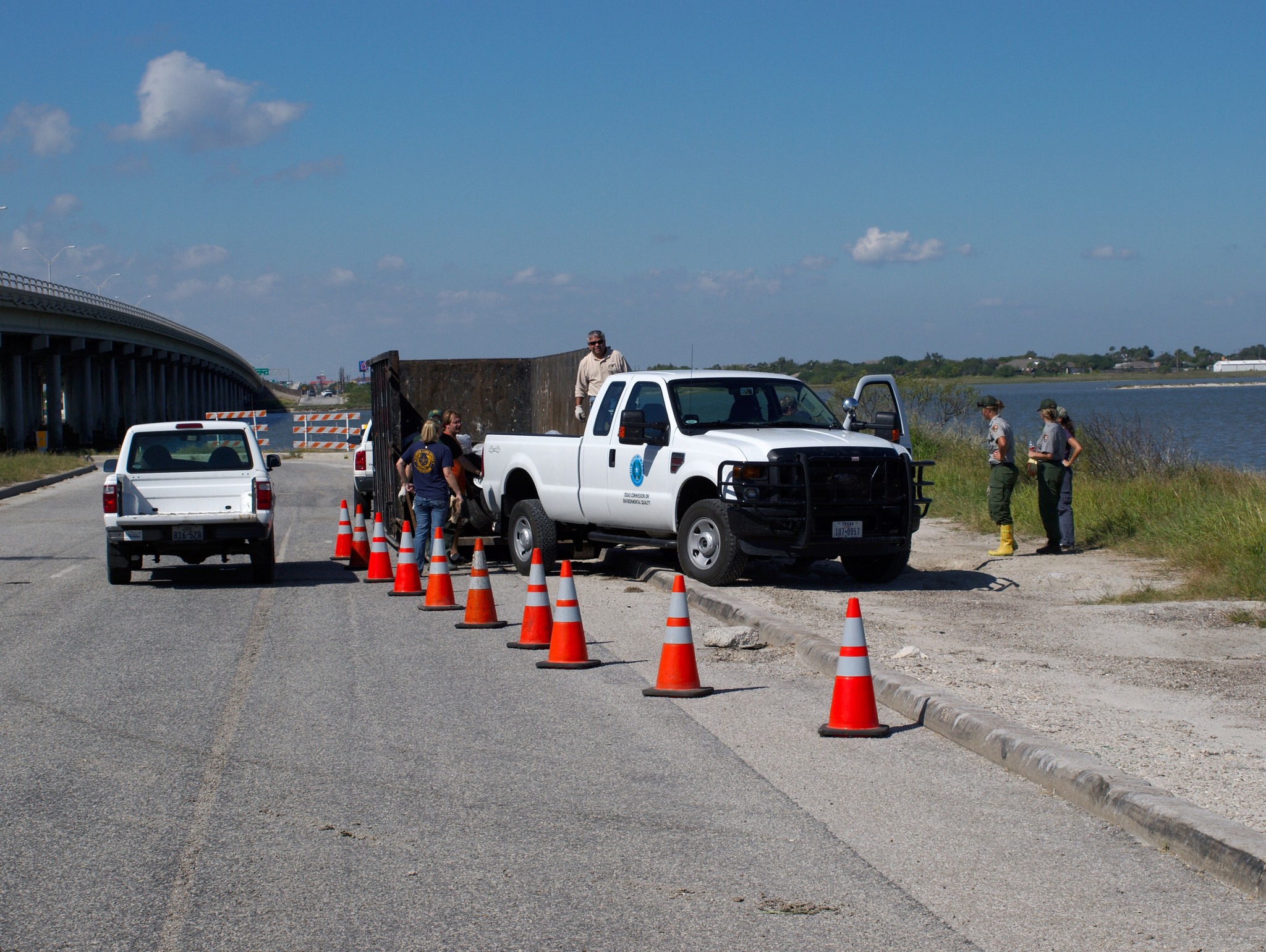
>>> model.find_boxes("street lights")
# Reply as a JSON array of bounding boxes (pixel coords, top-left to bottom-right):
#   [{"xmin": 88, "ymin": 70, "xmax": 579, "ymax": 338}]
[
  {"xmin": 75, "ymin": 274, "xmax": 121, "ymax": 298},
  {"xmin": 22, "ymin": 245, "xmax": 77, "ymax": 295},
  {"xmin": 304, "ymin": 367, "xmax": 313, "ymax": 384},
  {"xmin": 328, "ymin": 363, "xmax": 338, "ymax": 394},
  {"xmin": 249, "ymin": 354, "xmax": 272, "ymax": 368},
  {"xmin": 113, "ymin": 295, "xmax": 151, "ymax": 310}
]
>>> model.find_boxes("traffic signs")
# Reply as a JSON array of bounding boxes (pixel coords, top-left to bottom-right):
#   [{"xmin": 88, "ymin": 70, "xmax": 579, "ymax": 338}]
[{"xmin": 254, "ymin": 368, "xmax": 269, "ymax": 376}]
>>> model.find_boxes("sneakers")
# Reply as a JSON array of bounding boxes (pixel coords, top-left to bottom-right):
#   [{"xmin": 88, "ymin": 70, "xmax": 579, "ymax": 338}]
[
  {"xmin": 1059, "ymin": 543, "xmax": 1073, "ymax": 552},
  {"xmin": 1037, "ymin": 542, "xmax": 1063, "ymax": 555}
]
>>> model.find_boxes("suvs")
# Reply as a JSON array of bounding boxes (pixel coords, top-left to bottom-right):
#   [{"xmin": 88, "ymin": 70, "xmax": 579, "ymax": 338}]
[
  {"xmin": 346, "ymin": 416, "xmax": 375, "ymax": 520},
  {"xmin": 307, "ymin": 390, "xmax": 317, "ymax": 397},
  {"xmin": 321, "ymin": 388, "xmax": 333, "ymax": 397}
]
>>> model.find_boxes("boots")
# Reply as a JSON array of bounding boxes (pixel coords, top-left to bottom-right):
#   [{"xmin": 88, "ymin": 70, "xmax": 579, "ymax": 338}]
[{"xmin": 989, "ymin": 524, "xmax": 1018, "ymax": 556}]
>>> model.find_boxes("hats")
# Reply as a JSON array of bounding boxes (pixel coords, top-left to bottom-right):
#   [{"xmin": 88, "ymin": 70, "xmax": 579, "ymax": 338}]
[
  {"xmin": 1056, "ymin": 406, "xmax": 1068, "ymax": 418},
  {"xmin": 427, "ymin": 410, "xmax": 444, "ymax": 420},
  {"xmin": 979, "ymin": 395, "xmax": 998, "ymax": 407},
  {"xmin": 779, "ymin": 395, "xmax": 798, "ymax": 408},
  {"xmin": 1037, "ymin": 399, "xmax": 1057, "ymax": 411}
]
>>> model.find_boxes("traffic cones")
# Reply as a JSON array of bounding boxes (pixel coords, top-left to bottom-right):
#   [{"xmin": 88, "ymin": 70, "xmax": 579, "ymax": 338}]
[
  {"xmin": 816, "ymin": 597, "xmax": 890, "ymax": 737},
  {"xmin": 362, "ymin": 511, "xmax": 395, "ymax": 583},
  {"xmin": 642, "ymin": 574, "xmax": 714, "ymax": 698},
  {"xmin": 534, "ymin": 559, "xmax": 601, "ymax": 670},
  {"xmin": 417, "ymin": 526, "xmax": 464, "ymax": 610},
  {"xmin": 505, "ymin": 547, "xmax": 553, "ymax": 649},
  {"xmin": 329, "ymin": 499, "xmax": 354, "ymax": 560},
  {"xmin": 387, "ymin": 520, "xmax": 427, "ymax": 596},
  {"xmin": 343, "ymin": 504, "xmax": 371, "ymax": 571},
  {"xmin": 454, "ymin": 539, "xmax": 509, "ymax": 629}
]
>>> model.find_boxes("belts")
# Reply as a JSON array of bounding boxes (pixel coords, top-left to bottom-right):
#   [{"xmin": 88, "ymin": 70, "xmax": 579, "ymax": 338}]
[{"xmin": 991, "ymin": 462, "xmax": 1013, "ymax": 469}]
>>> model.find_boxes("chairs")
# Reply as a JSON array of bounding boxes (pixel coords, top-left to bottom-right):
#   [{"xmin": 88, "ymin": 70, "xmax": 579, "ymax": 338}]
[
  {"xmin": 642, "ymin": 403, "xmax": 670, "ymax": 438},
  {"xmin": 725, "ymin": 399, "xmax": 767, "ymax": 422},
  {"xmin": 207, "ymin": 446, "xmax": 243, "ymax": 468},
  {"xmin": 139, "ymin": 445, "xmax": 173, "ymax": 472}
]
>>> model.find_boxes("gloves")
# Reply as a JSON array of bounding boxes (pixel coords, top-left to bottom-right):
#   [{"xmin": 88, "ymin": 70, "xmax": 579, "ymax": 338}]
[{"xmin": 575, "ymin": 405, "xmax": 586, "ymax": 424}]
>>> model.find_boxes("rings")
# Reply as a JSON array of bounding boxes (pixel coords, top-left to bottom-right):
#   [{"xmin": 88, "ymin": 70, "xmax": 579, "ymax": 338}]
[{"xmin": 994, "ymin": 453, "xmax": 996, "ymax": 455}]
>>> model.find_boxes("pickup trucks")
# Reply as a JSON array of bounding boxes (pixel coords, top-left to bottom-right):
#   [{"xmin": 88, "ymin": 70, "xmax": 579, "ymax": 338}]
[
  {"xmin": 473, "ymin": 367, "xmax": 939, "ymax": 590},
  {"xmin": 102, "ymin": 418, "xmax": 283, "ymax": 586}
]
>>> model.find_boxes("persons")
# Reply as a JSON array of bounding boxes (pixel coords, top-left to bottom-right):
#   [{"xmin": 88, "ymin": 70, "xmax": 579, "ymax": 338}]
[
  {"xmin": 1056, "ymin": 406, "xmax": 1075, "ymax": 548},
  {"xmin": 574, "ymin": 331, "xmax": 637, "ymax": 436},
  {"xmin": 978, "ymin": 395, "xmax": 1018, "ymax": 557},
  {"xmin": 396, "ymin": 409, "xmax": 484, "ymax": 578},
  {"xmin": 1026, "ymin": 398, "xmax": 1065, "ymax": 553}
]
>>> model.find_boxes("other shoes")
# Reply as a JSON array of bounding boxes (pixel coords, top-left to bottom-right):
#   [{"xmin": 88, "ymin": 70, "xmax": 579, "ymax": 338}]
[
  {"xmin": 449, "ymin": 555, "xmax": 473, "ymax": 566},
  {"xmin": 448, "ymin": 560, "xmax": 457, "ymax": 571}
]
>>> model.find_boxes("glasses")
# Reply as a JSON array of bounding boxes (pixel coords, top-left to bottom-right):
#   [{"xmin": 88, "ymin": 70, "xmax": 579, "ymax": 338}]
[
  {"xmin": 977, "ymin": 402, "xmax": 987, "ymax": 407},
  {"xmin": 590, "ymin": 339, "xmax": 604, "ymax": 346}
]
